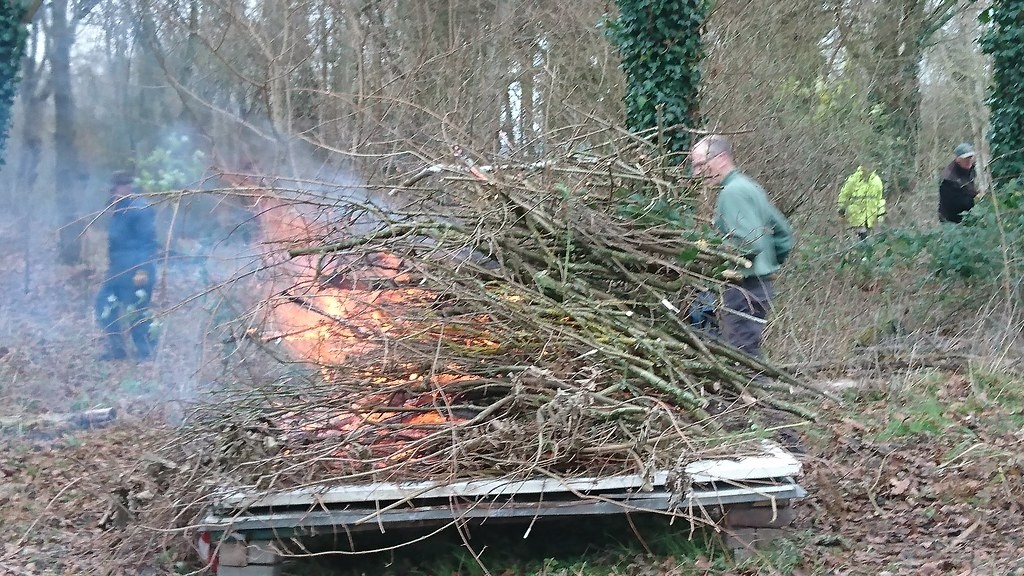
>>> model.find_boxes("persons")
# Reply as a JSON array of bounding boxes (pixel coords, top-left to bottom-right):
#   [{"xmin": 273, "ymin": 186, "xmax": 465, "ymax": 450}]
[
  {"xmin": 938, "ymin": 143, "xmax": 979, "ymax": 224},
  {"xmin": 94, "ymin": 172, "xmax": 157, "ymax": 362},
  {"xmin": 837, "ymin": 157, "xmax": 886, "ymax": 240},
  {"xmin": 687, "ymin": 133, "xmax": 791, "ymax": 408}
]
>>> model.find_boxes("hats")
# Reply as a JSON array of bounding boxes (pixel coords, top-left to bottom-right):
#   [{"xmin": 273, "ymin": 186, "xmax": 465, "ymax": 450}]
[
  {"xmin": 107, "ymin": 170, "xmax": 133, "ymax": 191},
  {"xmin": 955, "ymin": 143, "xmax": 975, "ymax": 160}
]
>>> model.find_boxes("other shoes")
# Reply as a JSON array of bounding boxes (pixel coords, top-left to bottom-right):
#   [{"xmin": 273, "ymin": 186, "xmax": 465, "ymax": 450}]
[{"xmin": 98, "ymin": 349, "xmax": 126, "ymax": 362}]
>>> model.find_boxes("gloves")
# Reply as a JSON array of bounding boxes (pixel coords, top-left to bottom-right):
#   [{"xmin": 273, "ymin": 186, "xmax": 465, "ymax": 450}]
[
  {"xmin": 134, "ymin": 270, "xmax": 148, "ymax": 287},
  {"xmin": 839, "ymin": 209, "xmax": 845, "ymax": 215}
]
